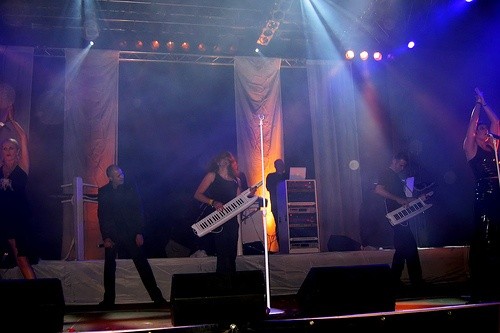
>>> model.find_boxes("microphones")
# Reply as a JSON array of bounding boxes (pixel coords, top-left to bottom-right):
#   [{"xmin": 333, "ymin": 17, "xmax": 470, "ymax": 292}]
[
  {"xmin": 97, "ymin": 242, "xmax": 116, "ymax": 248},
  {"xmin": 487, "ymin": 133, "xmax": 500, "ymax": 140}
]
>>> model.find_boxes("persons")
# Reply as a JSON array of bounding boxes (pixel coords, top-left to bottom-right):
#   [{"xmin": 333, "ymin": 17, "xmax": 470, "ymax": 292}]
[
  {"xmin": 463, "ymin": 87, "xmax": 500, "ymax": 246},
  {"xmin": 266, "ymin": 159, "xmax": 290, "ymax": 254},
  {"xmin": 0, "ymin": 104, "xmax": 45, "ymax": 280},
  {"xmin": 375, "ymin": 152, "xmax": 431, "ymax": 295},
  {"xmin": 99, "ymin": 165, "xmax": 167, "ymax": 304},
  {"xmin": 194, "ymin": 150, "xmax": 256, "ymax": 272}
]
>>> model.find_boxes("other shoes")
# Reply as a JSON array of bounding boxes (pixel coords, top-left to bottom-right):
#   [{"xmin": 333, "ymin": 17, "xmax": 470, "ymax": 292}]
[
  {"xmin": 94, "ymin": 301, "xmax": 115, "ymax": 310},
  {"xmin": 154, "ymin": 301, "xmax": 169, "ymax": 308},
  {"xmin": 414, "ymin": 280, "xmax": 432, "ymax": 288}
]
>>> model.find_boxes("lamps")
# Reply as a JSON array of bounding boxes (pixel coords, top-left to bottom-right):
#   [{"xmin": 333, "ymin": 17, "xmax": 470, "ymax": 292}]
[{"xmin": 257, "ymin": 0, "xmax": 290, "ymax": 45}]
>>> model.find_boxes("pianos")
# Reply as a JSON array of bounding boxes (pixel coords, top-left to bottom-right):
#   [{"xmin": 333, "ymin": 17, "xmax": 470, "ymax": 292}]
[
  {"xmin": 384, "ymin": 190, "xmax": 435, "ymax": 227},
  {"xmin": 191, "ymin": 181, "xmax": 265, "ymax": 237}
]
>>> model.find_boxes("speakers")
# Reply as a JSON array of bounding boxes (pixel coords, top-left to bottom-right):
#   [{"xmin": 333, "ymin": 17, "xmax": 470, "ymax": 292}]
[
  {"xmin": 170, "ymin": 269, "xmax": 267, "ymax": 326},
  {"xmin": 0, "ymin": 277, "xmax": 65, "ymax": 333},
  {"xmin": 294, "ymin": 263, "xmax": 396, "ymax": 323}
]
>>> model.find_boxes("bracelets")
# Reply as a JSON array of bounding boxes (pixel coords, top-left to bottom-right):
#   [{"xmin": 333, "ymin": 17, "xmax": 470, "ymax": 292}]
[
  {"xmin": 476, "ymin": 102, "xmax": 481, "ymax": 104},
  {"xmin": 212, "ymin": 200, "xmax": 216, "ymax": 207},
  {"xmin": 482, "ymin": 103, "xmax": 487, "ymax": 106}
]
